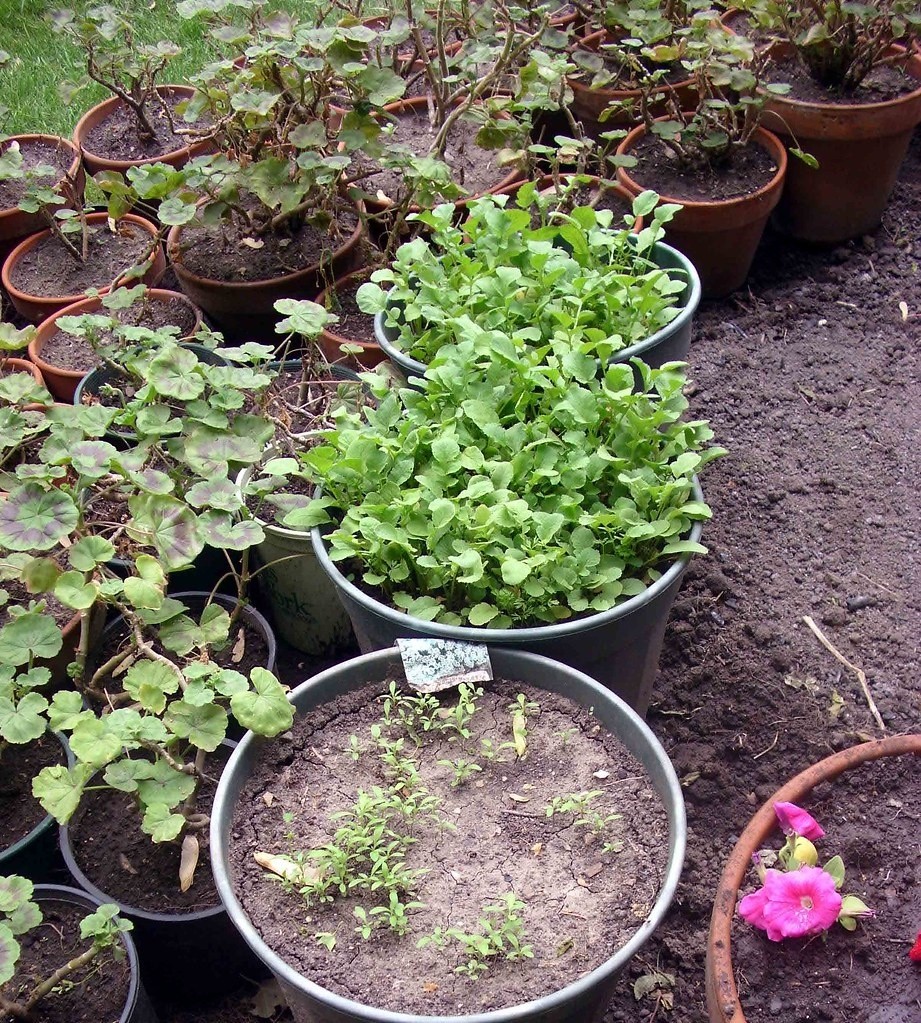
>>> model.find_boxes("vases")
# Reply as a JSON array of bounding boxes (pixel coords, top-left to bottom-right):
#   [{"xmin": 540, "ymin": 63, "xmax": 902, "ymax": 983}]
[{"xmin": 706, "ymin": 735, "xmax": 921, "ymax": 1023}]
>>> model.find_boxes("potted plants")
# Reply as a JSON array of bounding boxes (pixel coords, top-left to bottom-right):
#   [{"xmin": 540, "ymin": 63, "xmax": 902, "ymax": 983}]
[{"xmin": 0, "ymin": 0, "xmax": 921, "ymax": 1023}]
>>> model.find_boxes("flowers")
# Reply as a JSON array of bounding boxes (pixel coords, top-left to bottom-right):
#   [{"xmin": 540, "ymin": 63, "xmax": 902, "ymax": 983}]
[{"xmin": 738, "ymin": 800, "xmax": 876, "ymax": 942}]
